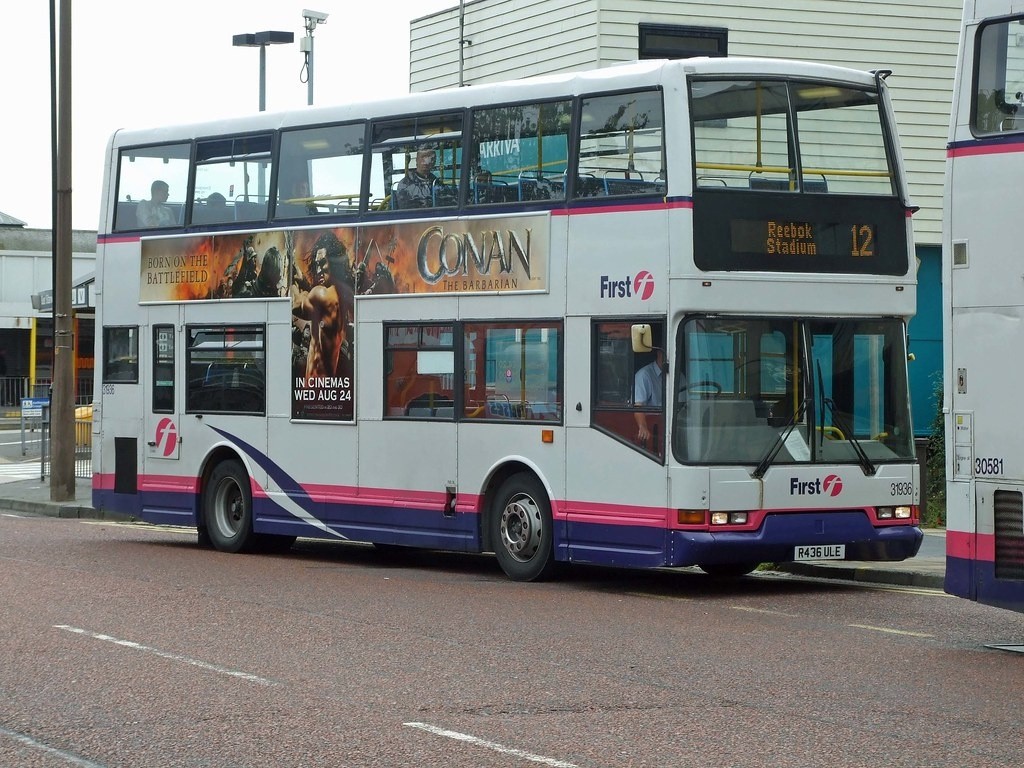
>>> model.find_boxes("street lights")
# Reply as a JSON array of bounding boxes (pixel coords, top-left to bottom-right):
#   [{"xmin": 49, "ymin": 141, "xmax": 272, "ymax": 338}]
[{"xmin": 231, "ymin": 30, "xmax": 298, "ymax": 114}]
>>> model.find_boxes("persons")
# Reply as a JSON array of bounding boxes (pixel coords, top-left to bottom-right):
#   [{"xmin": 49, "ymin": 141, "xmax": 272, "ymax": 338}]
[
  {"xmin": 361, "ymin": 262, "xmax": 395, "ymax": 294},
  {"xmin": 631, "ymin": 339, "xmax": 690, "ymax": 442},
  {"xmin": 476, "ymin": 171, "xmax": 503, "ymax": 203},
  {"xmin": 397, "ymin": 145, "xmax": 454, "ymax": 209},
  {"xmin": 232, "ymin": 248, "xmax": 257, "ymax": 294},
  {"xmin": 352, "ymin": 262, "xmax": 372, "ymax": 295},
  {"xmin": 253, "ymin": 247, "xmax": 294, "ymax": 297},
  {"xmin": 0, "ymin": 346, "xmax": 14, "ymax": 406},
  {"xmin": 291, "ymin": 233, "xmax": 352, "ymax": 389},
  {"xmin": 286, "ymin": 178, "xmax": 318, "ymax": 216},
  {"xmin": 134, "ymin": 180, "xmax": 178, "ymax": 229},
  {"xmin": 206, "ymin": 193, "xmax": 227, "ymax": 207}
]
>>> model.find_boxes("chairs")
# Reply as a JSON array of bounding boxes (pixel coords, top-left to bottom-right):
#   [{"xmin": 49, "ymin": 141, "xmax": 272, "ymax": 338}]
[{"xmin": 114, "ymin": 165, "xmax": 834, "ymax": 226}]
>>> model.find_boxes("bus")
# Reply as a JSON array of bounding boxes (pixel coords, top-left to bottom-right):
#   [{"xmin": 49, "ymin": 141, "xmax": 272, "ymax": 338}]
[
  {"xmin": 938, "ymin": 0, "xmax": 1024, "ymax": 616},
  {"xmin": 91, "ymin": 56, "xmax": 927, "ymax": 583}
]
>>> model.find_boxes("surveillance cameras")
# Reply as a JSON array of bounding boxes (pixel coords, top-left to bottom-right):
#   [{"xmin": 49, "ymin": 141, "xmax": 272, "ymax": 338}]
[{"xmin": 302, "ymin": 9, "xmax": 329, "ymax": 24}]
